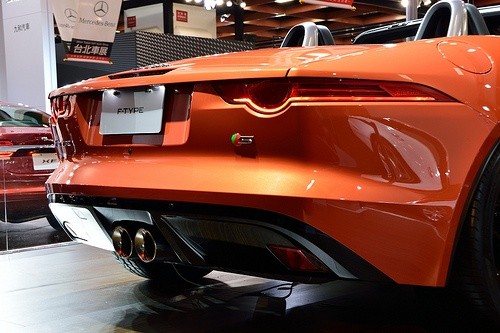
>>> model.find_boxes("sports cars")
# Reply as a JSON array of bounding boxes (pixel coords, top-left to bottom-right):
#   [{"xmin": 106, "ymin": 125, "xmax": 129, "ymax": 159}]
[{"xmin": 45, "ymin": 0, "xmax": 500, "ymax": 333}]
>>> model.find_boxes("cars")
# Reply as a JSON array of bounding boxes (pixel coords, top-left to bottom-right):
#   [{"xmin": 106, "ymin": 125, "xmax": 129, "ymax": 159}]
[{"xmin": 0, "ymin": 100, "xmax": 63, "ymax": 231}]
